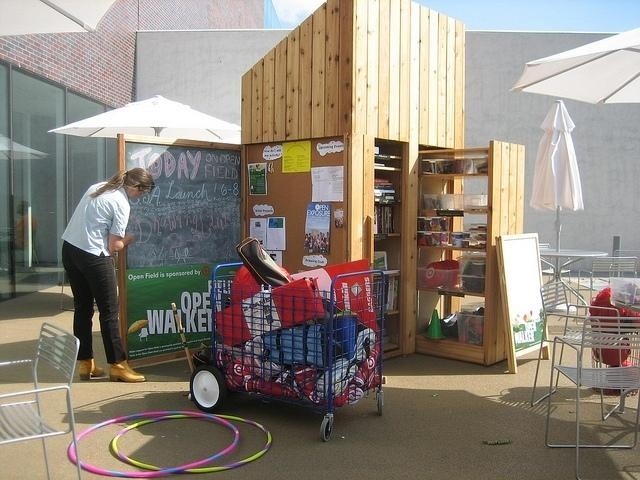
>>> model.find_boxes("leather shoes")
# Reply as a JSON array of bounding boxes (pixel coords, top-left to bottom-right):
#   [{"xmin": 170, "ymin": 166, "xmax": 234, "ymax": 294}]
[
  {"xmin": 77, "ymin": 358, "xmax": 105, "ymax": 380},
  {"xmin": 110, "ymin": 360, "xmax": 145, "ymax": 382}
]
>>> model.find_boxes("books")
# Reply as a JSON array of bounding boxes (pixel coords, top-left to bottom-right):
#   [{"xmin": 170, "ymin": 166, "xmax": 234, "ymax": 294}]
[{"xmin": 372, "ymin": 145, "xmax": 401, "ymax": 316}]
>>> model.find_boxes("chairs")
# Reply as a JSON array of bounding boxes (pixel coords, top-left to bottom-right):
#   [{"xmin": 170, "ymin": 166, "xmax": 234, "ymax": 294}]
[
  {"xmin": 0, "ymin": 323, "xmax": 82, "ymax": 480},
  {"xmin": 531, "ymin": 243, "xmax": 640, "ymax": 449}
]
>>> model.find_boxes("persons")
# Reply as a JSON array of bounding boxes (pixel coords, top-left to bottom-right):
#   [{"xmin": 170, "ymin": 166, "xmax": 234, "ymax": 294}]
[
  {"xmin": 305, "ymin": 232, "xmax": 329, "ymax": 253},
  {"xmin": 5, "ymin": 200, "xmax": 37, "ymax": 250},
  {"xmin": 58, "ymin": 167, "xmax": 156, "ymax": 382}
]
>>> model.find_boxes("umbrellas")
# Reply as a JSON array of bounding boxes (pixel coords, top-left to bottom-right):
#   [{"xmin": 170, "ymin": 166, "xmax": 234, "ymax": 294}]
[
  {"xmin": 0, "ymin": 134, "xmax": 50, "ymax": 161},
  {"xmin": 510, "ymin": 28, "xmax": 640, "ymax": 105},
  {"xmin": 0, "ymin": 0, "xmax": 116, "ymax": 36},
  {"xmin": 532, "ymin": 98, "xmax": 584, "ymax": 279},
  {"xmin": 47, "ymin": 93, "xmax": 242, "ymax": 143}
]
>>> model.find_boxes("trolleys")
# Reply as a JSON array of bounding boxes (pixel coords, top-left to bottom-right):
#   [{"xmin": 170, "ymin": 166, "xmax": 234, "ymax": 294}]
[{"xmin": 190, "ymin": 261, "xmax": 386, "ymax": 442}]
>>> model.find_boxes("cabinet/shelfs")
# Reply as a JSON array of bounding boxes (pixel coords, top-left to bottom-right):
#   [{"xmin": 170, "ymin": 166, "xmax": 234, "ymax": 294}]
[{"xmin": 373, "ymin": 137, "xmax": 526, "ymax": 368}]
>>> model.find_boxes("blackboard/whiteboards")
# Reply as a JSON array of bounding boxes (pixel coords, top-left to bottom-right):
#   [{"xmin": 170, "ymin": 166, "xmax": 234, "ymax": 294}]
[
  {"xmin": 498, "ymin": 233, "xmax": 549, "ymax": 359},
  {"xmin": 119, "ymin": 134, "xmax": 247, "ymax": 371}
]
